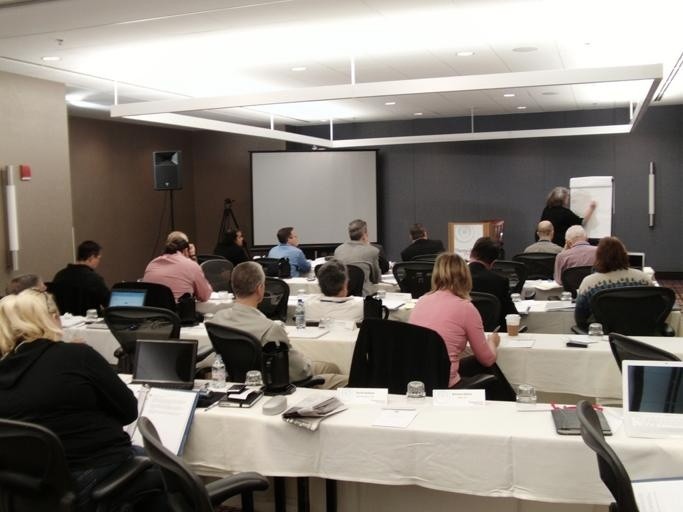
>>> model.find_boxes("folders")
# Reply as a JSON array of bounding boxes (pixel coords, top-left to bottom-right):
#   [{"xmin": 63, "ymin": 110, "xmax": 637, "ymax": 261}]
[{"xmin": 123, "ymin": 382, "xmax": 199, "ymax": 457}]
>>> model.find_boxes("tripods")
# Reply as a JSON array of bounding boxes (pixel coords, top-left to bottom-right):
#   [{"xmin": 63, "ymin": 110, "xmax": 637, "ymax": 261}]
[{"xmin": 216, "ymin": 208, "xmax": 249, "ymax": 253}]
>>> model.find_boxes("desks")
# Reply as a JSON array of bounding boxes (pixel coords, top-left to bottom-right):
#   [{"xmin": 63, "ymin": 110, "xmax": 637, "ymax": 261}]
[
  {"xmin": 113, "ymin": 372, "xmax": 683, "ymax": 511},
  {"xmin": 60, "ymin": 315, "xmax": 683, "ymax": 399},
  {"xmin": 192, "ymin": 290, "xmax": 683, "ymax": 336},
  {"xmin": 283, "ymin": 276, "xmax": 569, "ymax": 299}
]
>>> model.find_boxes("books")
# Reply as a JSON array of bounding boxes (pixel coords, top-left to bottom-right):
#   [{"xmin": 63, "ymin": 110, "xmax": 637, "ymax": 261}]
[
  {"xmin": 218, "ymin": 382, "xmax": 264, "ymax": 411},
  {"xmin": 551, "ymin": 406, "xmax": 612, "ymax": 436}
]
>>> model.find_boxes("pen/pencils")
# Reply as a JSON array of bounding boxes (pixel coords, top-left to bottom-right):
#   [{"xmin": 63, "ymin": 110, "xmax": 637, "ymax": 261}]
[
  {"xmin": 491, "ymin": 325, "xmax": 501, "ymax": 336},
  {"xmin": 203, "ymin": 400, "xmax": 219, "ymax": 411}
]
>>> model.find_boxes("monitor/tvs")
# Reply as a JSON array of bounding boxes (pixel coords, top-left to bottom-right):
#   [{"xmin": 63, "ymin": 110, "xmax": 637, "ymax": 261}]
[
  {"xmin": 108, "ymin": 290, "xmax": 146, "ymax": 308},
  {"xmin": 621, "ymin": 360, "xmax": 683, "ymax": 442},
  {"xmin": 627, "ymin": 251, "xmax": 645, "ymax": 272},
  {"xmin": 128, "ymin": 338, "xmax": 198, "ymax": 397}
]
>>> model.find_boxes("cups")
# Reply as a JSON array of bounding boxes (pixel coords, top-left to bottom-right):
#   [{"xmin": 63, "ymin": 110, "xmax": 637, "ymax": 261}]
[
  {"xmin": 516, "ymin": 383, "xmax": 537, "ymax": 412},
  {"xmin": 505, "ymin": 314, "xmax": 521, "ymax": 337},
  {"xmin": 376, "ymin": 290, "xmax": 387, "ymax": 300},
  {"xmin": 296, "ymin": 289, "xmax": 304, "ymax": 300},
  {"xmin": 204, "ymin": 313, "xmax": 215, "ymax": 323},
  {"xmin": 511, "ymin": 293, "xmax": 521, "ymax": 303},
  {"xmin": 561, "ymin": 292, "xmax": 572, "ymax": 307},
  {"xmin": 307, "ymin": 270, "xmax": 316, "ymax": 281},
  {"xmin": 406, "ymin": 381, "xmax": 426, "ymax": 410},
  {"xmin": 588, "ymin": 323, "xmax": 604, "ymax": 345},
  {"xmin": 85, "ymin": 309, "xmax": 98, "ymax": 325},
  {"xmin": 247, "ymin": 370, "xmax": 263, "ymax": 392}
]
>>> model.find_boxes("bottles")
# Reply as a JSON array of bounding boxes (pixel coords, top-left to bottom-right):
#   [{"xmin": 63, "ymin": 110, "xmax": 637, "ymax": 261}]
[
  {"xmin": 295, "ymin": 298, "xmax": 307, "ymax": 330},
  {"xmin": 211, "ymin": 354, "xmax": 227, "ymax": 390}
]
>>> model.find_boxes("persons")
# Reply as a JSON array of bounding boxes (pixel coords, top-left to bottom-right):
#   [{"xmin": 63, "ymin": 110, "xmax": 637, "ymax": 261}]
[
  {"xmin": 535, "ymin": 186, "xmax": 596, "ymax": 247},
  {"xmin": 555, "ymin": 224, "xmax": 600, "ymax": 285},
  {"xmin": 401, "ymin": 222, "xmax": 445, "ymax": 260},
  {"xmin": 213, "ymin": 229, "xmax": 250, "ymax": 266},
  {"xmin": 143, "ymin": 232, "xmax": 212, "ymax": 303},
  {"xmin": 576, "ymin": 238, "xmax": 655, "ymax": 330},
  {"xmin": 4, "ymin": 274, "xmax": 47, "ymax": 295},
  {"xmin": 267, "ymin": 227, "xmax": 309, "ymax": 277},
  {"xmin": 333, "ymin": 220, "xmax": 382, "ymax": 296},
  {"xmin": 54, "ymin": 241, "xmax": 110, "ymax": 315},
  {"xmin": 408, "ymin": 254, "xmax": 516, "ymax": 400},
  {"xmin": 209, "ymin": 262, "xmax": 349, "ymax": 391},
  {"xmin": 308, "ymin": 260, "xmax": 364, "ymax": 325},
  {"xmin": 464, "ymin": 237, "xmax": 519, "ymax": 318},
  {"xmin": 525, "ymin": 221, "xmax": 563, "ymax": 254},
  {"xmin": 0, "ymin": 288, "xmax": 161, "ymax": 511}
]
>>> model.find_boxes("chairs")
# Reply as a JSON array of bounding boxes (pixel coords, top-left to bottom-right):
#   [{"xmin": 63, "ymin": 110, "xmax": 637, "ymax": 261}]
[
  {"xmin": 136, "ymin": 415, "xmax": 269, "ymax": 511},
  {"xmin": 411, "ymin": 254, "xmax": 439, "ymax": 262},
  {"xmin": 197, "ymin": 254, "xmax": 225, "ymax": 265},
  {"xmin": 314, "ymin": 264, "xmax": 365, "ymax": 296},
  {"xmin": 571, "ymin": 285, "xmax": 676, "ymax": 337},
  {"xmin": 103, "ymin": 307, "xmax": 215, "ymax": 375},
  {"xmin": 575, "ymin": 401, "xmax": 638, "ymax": 511},
  {"xmin": 205, "ymin": 321, "xmax": 325, "ymax": 388},
  {"xmin": 609, "ymin": 333, "xmax": 681, "ymax": 373},
  {"xmin": 110, "ymin": 282, "xmax": 176, "ymax": 310},
  {"xmin": 561, "ymin": 266, "xmax": 595, "ymax": 299},
  {"xmin": 449, "ymin": 374, "xmax": 497, "ymax": 401},
  {"xmin": 200, "ymin": 259, "xmax": 233, "ymax": 293},
  {"xmin": 469, "ymin": 292, "xmax": 500, "ymax": 332},
  {"xmin": 257, "ymin": 277, "xmax": 290, "ymax": 323},
  {"xmin": 492, "ymin": 261, "xmax": 528, "ymax": 293},
  {"xmin": 392, "ymin": 262, "xmax": 435, "ymax": 299},
  {"xmin": 513, "ymin": 252, "xmax": 557, "ymax": 280},
  {"xmin": 0, "ymin": 417, "xmax": 152, "ymax": 512}
]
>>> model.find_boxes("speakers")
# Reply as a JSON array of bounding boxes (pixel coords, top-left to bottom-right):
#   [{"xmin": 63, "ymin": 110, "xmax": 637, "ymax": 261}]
[{"xmin": 152, "ymin": 149, "xmax": 183, "ymax": 190}]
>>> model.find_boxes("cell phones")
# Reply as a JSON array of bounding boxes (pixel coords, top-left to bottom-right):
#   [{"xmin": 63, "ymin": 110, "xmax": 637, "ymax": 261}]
[{"xmin": 227, "ymin": 385, "xmax": 246, "ymax": 393}]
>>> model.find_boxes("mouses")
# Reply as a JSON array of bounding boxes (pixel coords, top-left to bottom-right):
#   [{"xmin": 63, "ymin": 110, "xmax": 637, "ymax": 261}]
[{"xmin": 199, "ymin": 389, "xmax": 213, "ymax": 399}]
